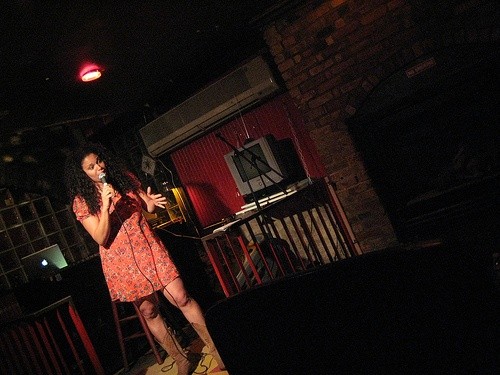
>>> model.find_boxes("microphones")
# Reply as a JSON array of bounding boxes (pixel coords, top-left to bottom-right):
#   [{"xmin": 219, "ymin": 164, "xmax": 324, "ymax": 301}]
[
  {"xmin": 215, "ymin": 134, "xmax": 240, "ymax": 154},
  {"xmin": 99, "ymin": 173, "xmax": 113, "ymax": 202}
]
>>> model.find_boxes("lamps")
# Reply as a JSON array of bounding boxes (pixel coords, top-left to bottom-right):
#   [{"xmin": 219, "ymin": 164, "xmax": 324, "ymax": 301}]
[{"xmin": 79, "ymin": 61, "xmax": 101, "ymax": 82}]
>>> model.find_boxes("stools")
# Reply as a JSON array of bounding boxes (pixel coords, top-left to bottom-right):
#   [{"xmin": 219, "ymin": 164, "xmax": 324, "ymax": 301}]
[{"xmin": 112, "ymin": 301, "xmax": 188, "ymax": 372}]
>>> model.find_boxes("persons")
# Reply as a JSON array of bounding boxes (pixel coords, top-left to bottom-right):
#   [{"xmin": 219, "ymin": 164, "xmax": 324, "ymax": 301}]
[{"xmin": 64, "ymin": 142, "xmax": 227, "ymax": 375}]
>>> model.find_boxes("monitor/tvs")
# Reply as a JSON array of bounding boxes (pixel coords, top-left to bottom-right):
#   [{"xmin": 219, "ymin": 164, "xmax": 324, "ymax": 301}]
[{"xmin": 224, "ymin": 135, "xmax": 289, "ymax": 195}]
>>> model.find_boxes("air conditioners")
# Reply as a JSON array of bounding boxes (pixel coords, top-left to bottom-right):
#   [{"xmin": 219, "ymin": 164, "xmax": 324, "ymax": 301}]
[{"xmin": 139, "ymin": 56, "xmax": 284, "ymax": 159}]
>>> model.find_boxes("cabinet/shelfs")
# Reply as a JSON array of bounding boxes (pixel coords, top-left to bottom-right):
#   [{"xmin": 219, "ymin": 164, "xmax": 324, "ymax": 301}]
[
  {"xmin": 111, "ymin": 122, "xmax": 177, "ymax": 193},
  {"xmin": 0, "ymin": 183, "xmax": 100, "ymax": 298}
]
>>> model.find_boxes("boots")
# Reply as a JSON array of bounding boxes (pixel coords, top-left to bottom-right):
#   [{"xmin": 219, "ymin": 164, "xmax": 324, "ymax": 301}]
[
  {"xmin": 152, "ymin": 326, "xmax": 191, "ymax": 375},
  {"xmin": 191, "ymin": 321, "xmax": 228, "ymax": 370}
]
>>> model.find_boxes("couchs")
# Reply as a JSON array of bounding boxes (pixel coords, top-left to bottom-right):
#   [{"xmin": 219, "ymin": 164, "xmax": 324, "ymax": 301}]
[{"xmin": 205, "ymin": 239, "xmax": 500, "ymax": 375}]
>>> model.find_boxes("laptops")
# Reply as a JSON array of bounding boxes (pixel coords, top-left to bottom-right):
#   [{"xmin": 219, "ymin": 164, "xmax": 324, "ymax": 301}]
[{"xmin": 22, "ymin": 244, "xmax": 68, "ymax": 280}]
